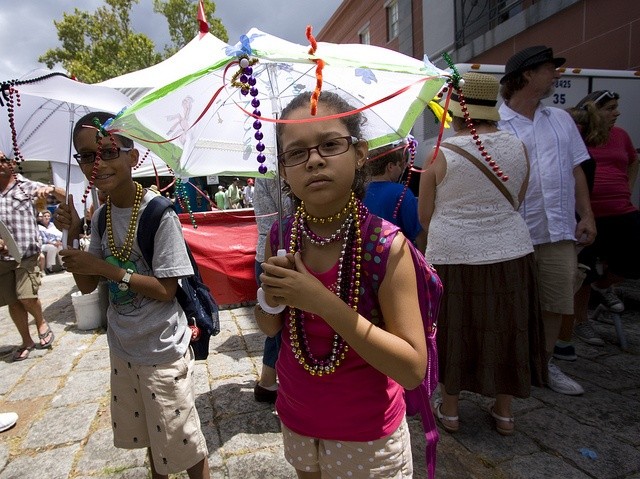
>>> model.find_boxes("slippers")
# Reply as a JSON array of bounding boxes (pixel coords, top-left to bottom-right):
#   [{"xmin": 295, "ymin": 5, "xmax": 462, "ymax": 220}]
[
  {"xmin": 38, "ymin": 322, "xmax": 55, "ymax": 349},
  {"xmin": 13, "ymin": 343, "xmax": 36, "ymax": 361}
]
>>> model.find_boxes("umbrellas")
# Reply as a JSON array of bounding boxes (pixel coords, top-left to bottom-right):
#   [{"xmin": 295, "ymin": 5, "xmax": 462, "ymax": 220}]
[
  {"xmin": 0, "ymin": 68, "xmax": 177, "ymax": 272},
  {"xmin": 102, "ymin": 42, "xmax": 450, "ymax": 257}
]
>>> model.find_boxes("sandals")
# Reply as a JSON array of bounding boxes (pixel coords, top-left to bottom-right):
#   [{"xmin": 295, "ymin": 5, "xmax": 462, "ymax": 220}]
[
  {"xmin": 432, "ymin": 397, "xmax": 461, "ymax": 434},
  {"xmin": 487, "ymin": 399, "xmax": 516, "ymax": 436}
]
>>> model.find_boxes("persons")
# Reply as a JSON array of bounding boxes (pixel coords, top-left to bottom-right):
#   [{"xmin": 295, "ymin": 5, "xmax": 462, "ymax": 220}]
[
  {"xmin": 37, "ymin": 210, "xmax": 68, "ymax": 273},
  {"xmin": 53, "ymin": 111, "xmax": 208, "ymax": 455},
  {"xmin": 255, "ymin": 93, "xmax": 428, "ymax": 479},
  {"xmin": 566, "ymin": 101, "xmax": 608, "ymax": 222},
  {"xmin": 242, "ymin": 178, "xmax": 254, "ymax": 208},
  {"xmin": 37, "ymin": 212, "xmax": 46, "ymax": 278},
  {"xmin": 418, "ymin": 73, "xmax": 546, "ymax": 437},
  {"xmin": 0, "ymin": 151, "xmax": 66, "ymax": 361},
  {"xmin": 229, "ymin": 177, "xmax": 242, "ymax": 211},
  {"xmin": 499, "ymin": 44, "xmax": 597, "ymax": 395},
  {"xmin": 214, "ymin": 185, "xmax": 226, "ymax": 209},
  {"xmin": 571, "ymin": 91, "xmax": 640, "ymax": 342}
]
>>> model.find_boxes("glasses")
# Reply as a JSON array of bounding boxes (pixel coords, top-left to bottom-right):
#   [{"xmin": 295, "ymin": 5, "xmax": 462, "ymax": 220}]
[
  {"xmin": 72, "ymin": 147, "xmax": 134, "ymax": 164},
  {"xmin": 0, "ymin": 156, "xmax": 9, "ymax": 164},
  {"xmin": 276, "ymin": 134, "xmax": 359, "ymax": 168}
]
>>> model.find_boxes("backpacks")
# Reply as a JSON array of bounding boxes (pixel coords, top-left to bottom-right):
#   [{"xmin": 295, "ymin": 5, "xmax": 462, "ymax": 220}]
[
  {"xmin": 96, "ymin": 192, "xmax": 220, "ymax": 362},
  {"xmin": 269, "ymin": 210, "xmax": 443, "ymax": 479}
]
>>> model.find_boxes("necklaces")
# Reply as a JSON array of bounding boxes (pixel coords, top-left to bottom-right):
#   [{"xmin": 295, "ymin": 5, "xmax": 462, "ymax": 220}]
[
  {"xmin": 106, "ymin": 181, "xmax": 142, "ymax": 263},
  {"xmin": 289, "ymin": 189, "xmax": 368, "ymax": 377}
]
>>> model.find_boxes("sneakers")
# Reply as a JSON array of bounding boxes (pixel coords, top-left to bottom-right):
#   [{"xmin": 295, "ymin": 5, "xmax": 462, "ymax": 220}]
[
  {"xmin": 552, "ymin": 344, "xmax": 579, "ymax": 362},
  {"xmin": 574, "ymin": 323, "xmax": 606, "ymax": 347},
  {"xmin": 602, "ymin": 292, "xmax": 625, "ymax": 313},
  {"xmin": 546, "ymin": 362, "xmax": 585, "ymax": 397}
]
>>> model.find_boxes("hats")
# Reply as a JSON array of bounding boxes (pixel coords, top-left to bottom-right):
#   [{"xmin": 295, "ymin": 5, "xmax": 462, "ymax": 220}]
[
  {"xmin": 218, "ymin": 186, "xmax": 225, "ymax": 190},
  {"xmin": 500, "ymin": 45, "xmax": 566, "ymax": 86},
  {"xmin": 232, "ymin": 177, "xmax": 240, "ymax": 182},
  {"xmin": 247, "ymin": 178, "xmax": 254, "ymax": 184},
  {"xmin": 439, "ymin": 72, "xmax": 502, "ymax": 122}
]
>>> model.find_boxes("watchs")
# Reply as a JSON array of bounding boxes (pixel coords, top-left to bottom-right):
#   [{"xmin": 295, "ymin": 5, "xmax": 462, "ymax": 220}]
[{"xmin": 117, "ymin": 267, "xmax": 133, "ymax": 292}]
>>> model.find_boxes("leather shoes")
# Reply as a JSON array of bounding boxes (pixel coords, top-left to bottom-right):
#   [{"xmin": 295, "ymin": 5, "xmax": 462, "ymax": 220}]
[{"xmin": 254, "ymin": 381, "xmax": 277, "ymax": 402}]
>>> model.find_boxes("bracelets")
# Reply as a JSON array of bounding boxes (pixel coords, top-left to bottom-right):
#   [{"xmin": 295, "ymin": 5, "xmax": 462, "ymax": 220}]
[{"xmin": 48, "ymin": 184, "xmax": 56, "ymax": 194}]
